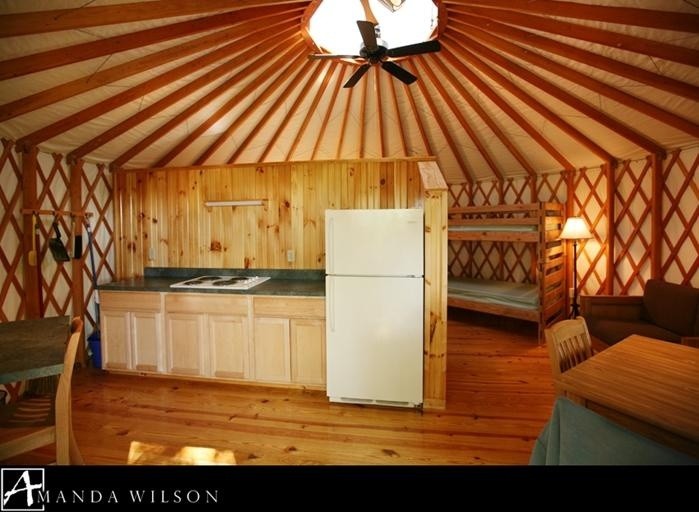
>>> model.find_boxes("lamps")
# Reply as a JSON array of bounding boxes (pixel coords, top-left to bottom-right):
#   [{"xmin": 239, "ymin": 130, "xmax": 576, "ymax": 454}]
[{"xmin": 558, "ymin": 216, "xmax": 595, "ymax": 318}]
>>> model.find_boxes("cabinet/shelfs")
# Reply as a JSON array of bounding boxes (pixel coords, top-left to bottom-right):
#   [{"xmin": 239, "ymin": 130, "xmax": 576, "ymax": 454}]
[
  {"xmin": 250, "ymin": 293, "xmax": 327, "ymax": 388},
  {"xmin": 96, "ymin": 290, "xmax": 166, "ymax": 376},
  {"xmin": 163, "ymin": 291, "xmax": 249, "ymax": 384}
]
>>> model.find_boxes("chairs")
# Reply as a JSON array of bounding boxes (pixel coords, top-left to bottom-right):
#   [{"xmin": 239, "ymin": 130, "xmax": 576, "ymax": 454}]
[
  {"xmin": 543, "ymin": 315, "xmax": 596, "ymax": 401},
  {"xmin": 578, "ymin": 278, "xmax": 698, "ymax": 354},
  {"xmin": 1, "ymin": 311, "xmax": 89, "ymax": 466}
]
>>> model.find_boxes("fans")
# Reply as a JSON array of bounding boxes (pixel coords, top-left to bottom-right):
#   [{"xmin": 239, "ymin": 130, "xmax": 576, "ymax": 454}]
[{"xmin": 304, "ymin": 18, "xmax": 443, "ymax": 89}]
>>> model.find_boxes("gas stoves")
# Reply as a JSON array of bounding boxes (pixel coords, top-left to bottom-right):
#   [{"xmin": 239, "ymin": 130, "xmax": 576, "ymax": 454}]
[{"xmin": 170, "ymin": 275, "xmax": 272, "ymax": 292}]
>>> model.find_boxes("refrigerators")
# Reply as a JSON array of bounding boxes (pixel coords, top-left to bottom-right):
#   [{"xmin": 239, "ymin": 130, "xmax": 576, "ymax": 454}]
[{"xmin": 324, "ymin": 208, "xmax": 426, "ymax": 410}]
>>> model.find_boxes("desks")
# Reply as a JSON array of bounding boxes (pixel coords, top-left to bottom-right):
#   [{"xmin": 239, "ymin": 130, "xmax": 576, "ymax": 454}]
[
  {"xmin": 526, "ymin": 332, "xmax": 698, "ymax": 467},
  {"xmin": 0, "ymin": 314, "xmax": 72, "ymax": 419}
]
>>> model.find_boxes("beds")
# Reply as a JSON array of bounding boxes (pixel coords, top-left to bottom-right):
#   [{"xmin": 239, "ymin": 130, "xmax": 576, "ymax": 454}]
[{"xmin": 447, "ymin": 199, "xmax": 567, "ymax": 345}]
